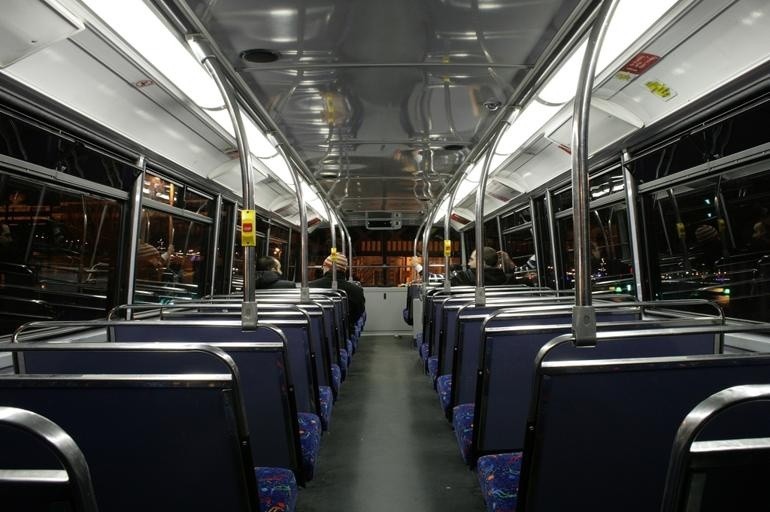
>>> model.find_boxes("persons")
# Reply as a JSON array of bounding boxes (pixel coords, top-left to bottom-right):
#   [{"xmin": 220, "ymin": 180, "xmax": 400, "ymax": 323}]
[
  {"xmin": 427, "ymin": 246, "xmax": 506, "ymax": 287},
  {"xmin": 306, "ymin": 251, "xmax": 366, "ymax": 339},
  {"xmin": 496, "ymin": 248, "xmax": 538, "ymax": 277},
  {"xmin": 746, "ymin": 220, "xmax": 769, "ymax": 254},
  {"xmin": 587, "ymin": 227, "xmax": 616, "ymax": 278},
  {"xmin": 409, "ymin": 254, "xmax": 463, "ymax": 283},
  {"xmin": 134, "ymin": 238, "xmax": 163, "ymax": 283},
  {"xmin": 253, "ymin": 255, "xmax": 296, "ymax": 288},
  {"xmin": 0, "ymin": 223, "xmax": 14, "ymax": 249}
]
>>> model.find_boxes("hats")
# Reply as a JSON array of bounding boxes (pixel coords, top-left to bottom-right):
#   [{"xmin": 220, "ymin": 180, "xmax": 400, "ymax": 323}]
[{"xmin": 323, "ymin": 253, "xmax": 347, "ymax": 271}]
